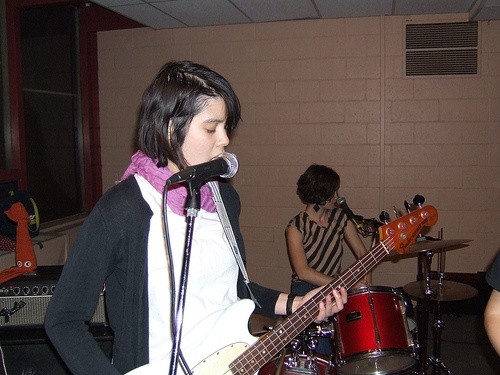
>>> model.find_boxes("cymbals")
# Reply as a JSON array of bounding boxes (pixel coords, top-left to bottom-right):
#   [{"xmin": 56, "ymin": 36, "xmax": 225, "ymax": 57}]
[
  {"xmin": 383, "ymin": 238, "xmax": 474, "ymax": 261},
  {"xmin": 403, "ymin": 278, "xmax": 479, "ymax": 301}
]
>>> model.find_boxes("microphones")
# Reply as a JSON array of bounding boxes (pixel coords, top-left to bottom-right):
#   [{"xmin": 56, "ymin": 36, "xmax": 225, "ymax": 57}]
[
  {"xmin": 166, "ymin": 152, "xmax": 239, "ymax": 186},
  {"xmin": 337, "ymin": 196, "xmax": 362, "ymax": 230}
]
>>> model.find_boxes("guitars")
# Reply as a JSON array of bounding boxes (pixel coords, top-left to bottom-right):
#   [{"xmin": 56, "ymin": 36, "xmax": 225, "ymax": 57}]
[{"xmin": 174, "ymin": 193, "xmax": 439, "ymax": 375}]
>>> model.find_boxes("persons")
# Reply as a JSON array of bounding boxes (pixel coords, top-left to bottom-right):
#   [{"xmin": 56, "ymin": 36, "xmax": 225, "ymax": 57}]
[
  {"xmin": 482, "ymin": 248, "xmax": 500, "ymax": 357},
  {"xmin": 43, "ymin": 59, "xmax": 348, "ymax": 375},
  {"xmin": 284, "ymin": 163, "xmax": 373, "ymax": 356}
]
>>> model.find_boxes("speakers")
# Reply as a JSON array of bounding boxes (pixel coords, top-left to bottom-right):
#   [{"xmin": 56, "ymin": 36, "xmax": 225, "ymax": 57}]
[{"xmin": 0, "ymin": 265, "xmax": 114, "ymax": 375}]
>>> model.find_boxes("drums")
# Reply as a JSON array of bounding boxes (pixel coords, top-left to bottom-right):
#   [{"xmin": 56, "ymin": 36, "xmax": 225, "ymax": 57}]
[
  {"xmin": 334, "ymin": 285, "xmax": 419, "ymax": 375},
  {"xmin": 257, "ymin": 353, "xmax": 336, "ymax": 375}
]
